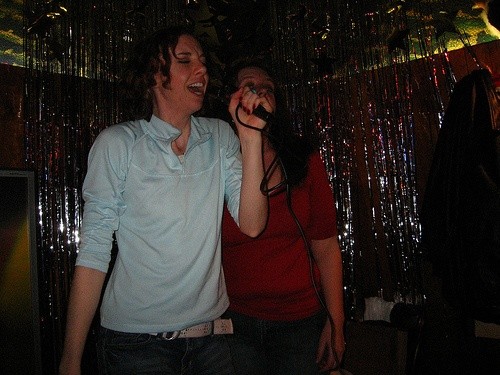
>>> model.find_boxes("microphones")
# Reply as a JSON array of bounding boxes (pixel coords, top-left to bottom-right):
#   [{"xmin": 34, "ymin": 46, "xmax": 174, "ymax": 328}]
[{"xmin": 218, "ymin": 85, "xmax": 274, "ymax": 124}]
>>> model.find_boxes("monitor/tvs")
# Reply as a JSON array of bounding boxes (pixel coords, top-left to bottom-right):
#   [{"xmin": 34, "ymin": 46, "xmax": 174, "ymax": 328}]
[{"xmin": 0, "ymin": 170, "xmax": 43, "ymax": 375}]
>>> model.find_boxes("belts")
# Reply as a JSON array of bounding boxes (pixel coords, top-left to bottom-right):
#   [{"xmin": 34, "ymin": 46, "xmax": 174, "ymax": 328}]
[{"xmin": 149, "ymin": 318, "xmax": 235, "ymax": 340}]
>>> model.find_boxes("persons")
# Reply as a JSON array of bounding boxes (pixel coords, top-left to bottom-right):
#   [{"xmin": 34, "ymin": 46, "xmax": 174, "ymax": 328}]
[
  {"xmin": 220, "ymin": 59, "xmax": 346, "ymax": 374},
  {"xmin": 57, "ymin": 25, "xmax": 273, "ymax": 375}
]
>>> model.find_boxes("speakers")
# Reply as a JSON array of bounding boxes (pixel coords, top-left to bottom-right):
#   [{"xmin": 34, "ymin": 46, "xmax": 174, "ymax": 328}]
[{"xmin": 339, "ymin": 322, "xmax": 415, "ymax": 375}]
